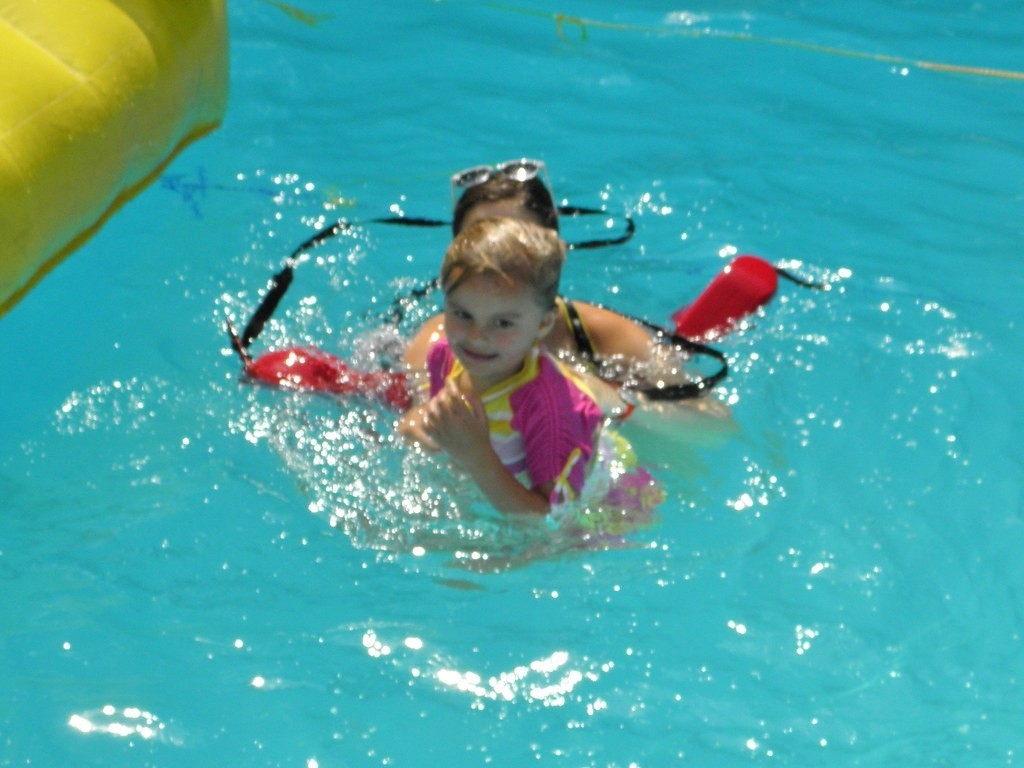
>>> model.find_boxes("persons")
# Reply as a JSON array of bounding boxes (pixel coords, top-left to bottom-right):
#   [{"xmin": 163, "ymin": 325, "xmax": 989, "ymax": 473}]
[
  {"xmin": 421, "ymin": 219, "xmax": 663, "ymax": 547},
  {"xmin": 407, "ymin": 158, "xmax": 733, "ymax": 452}
]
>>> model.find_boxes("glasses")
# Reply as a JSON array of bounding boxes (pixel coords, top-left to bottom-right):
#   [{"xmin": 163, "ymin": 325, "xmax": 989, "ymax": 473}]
[{"xmin": 448, "ymin": 157, "xmax": 557, "ymax": 213}]
